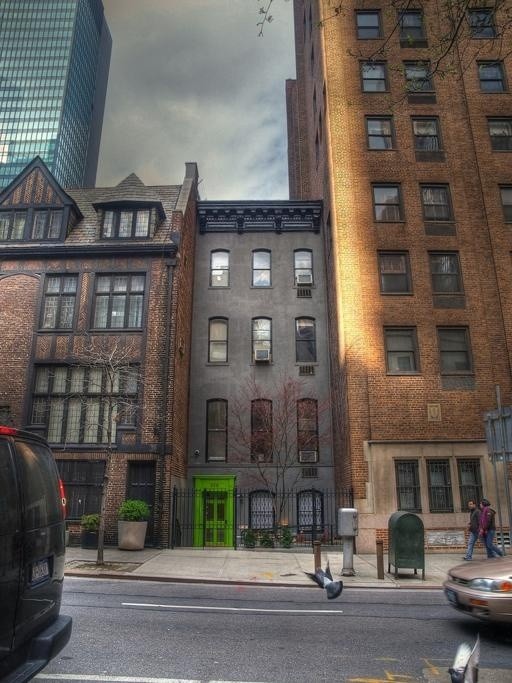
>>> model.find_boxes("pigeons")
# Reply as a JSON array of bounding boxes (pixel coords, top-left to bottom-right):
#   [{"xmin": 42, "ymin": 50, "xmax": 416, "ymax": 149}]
[
  {"xmin": 444, "ymin": 632, "xmax": 481, "ymax": 683},
  {"xmin": 302, "ymin": 559, "xmax": 344, "ymax": 600}
]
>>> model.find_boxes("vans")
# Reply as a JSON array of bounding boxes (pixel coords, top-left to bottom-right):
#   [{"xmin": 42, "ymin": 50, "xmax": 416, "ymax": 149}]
[{"xmin": 0, "ymin": 425, "xmax": 74, "ymax": 682}]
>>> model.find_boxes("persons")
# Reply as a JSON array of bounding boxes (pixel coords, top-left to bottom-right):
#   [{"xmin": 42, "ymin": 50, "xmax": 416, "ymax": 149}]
[
  {"xmin": 477, "ymin": 498, "xmax": 504, "ymax": 561},
  {"xmin": 461, "ymin": 498, "xmax": 482, "ymax": 561}
]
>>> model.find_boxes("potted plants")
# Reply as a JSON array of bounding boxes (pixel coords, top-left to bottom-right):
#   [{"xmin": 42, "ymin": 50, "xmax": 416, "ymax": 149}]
[{"xmin": 79, "ymin": 497, "xmax": 151, "ymax": 551}]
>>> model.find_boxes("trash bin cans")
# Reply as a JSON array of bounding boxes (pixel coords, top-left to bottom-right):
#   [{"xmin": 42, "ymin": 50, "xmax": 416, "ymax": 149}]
[{"xmin": 388, "ymin": 511, "xmax": 426, "ymax": 581}]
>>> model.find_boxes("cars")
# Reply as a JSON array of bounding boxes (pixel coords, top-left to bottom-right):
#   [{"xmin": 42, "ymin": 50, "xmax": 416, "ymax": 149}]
[{"xmin": 443, "ymin": 558, "xmax": 512, "ymax": 623}]
